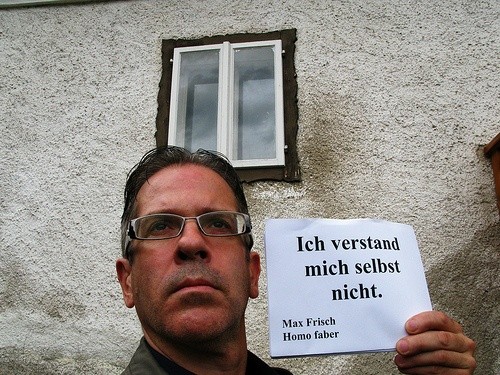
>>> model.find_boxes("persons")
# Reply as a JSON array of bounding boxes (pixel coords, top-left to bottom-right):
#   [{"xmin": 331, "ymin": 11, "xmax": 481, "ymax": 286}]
[{"xmin": 117, "ymin": 144, "xmax": 476, "ymax": 375}]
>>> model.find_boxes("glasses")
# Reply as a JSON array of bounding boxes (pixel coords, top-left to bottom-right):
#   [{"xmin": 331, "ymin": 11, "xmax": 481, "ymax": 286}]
[{"xmin": 122, "ymin": 211, "xmax": 253, "ymax": 260}]
